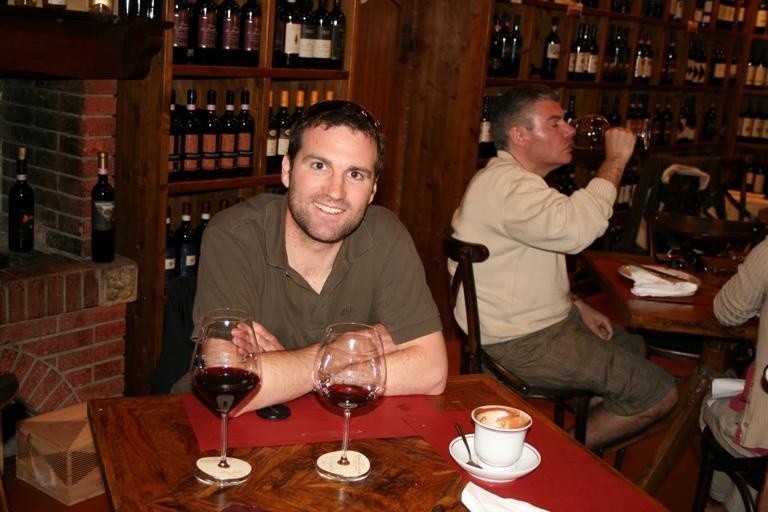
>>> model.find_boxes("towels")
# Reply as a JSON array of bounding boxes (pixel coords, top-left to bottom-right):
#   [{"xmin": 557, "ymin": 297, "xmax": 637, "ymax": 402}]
[
  {"xmin": 694, "ymin": 378, "xmax": 745, "ymax": 432},
  {"xmin": 449, "ymin": 477, "xmax": 550, "ymax": 512}
]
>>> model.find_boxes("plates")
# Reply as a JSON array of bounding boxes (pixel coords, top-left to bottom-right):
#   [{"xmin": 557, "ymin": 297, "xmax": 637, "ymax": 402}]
[
  {"xmin": 446, "ymin": 432, "xmax": 543, "ymax": 484},
  {"xmin": 618, "ymin": 263, "xmax": 703, "ymax": 290}
]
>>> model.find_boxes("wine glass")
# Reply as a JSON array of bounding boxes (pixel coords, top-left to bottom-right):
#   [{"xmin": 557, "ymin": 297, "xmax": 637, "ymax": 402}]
[
  {"xmin": 568, "ymin": 114, "xmax": 652, "ymax": 152},
  {"xmin": 187, "ymin": 306, "xmax": 263, "ymax": 489},
  {"xmin": 310, "ymin": 322, "xmax": 388, "ymax": 483}
]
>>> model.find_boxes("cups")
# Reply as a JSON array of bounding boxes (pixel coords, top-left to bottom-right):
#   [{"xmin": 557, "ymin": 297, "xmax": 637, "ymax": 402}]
[{"xmin": 471, "ymin": 404, "xmax": 535, "ymax": 470}]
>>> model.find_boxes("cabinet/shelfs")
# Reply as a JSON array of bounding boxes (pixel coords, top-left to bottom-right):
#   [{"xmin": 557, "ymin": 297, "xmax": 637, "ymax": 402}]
[
  {"xmin": 117, "ymin": 0, "xmax": 354, "ymax": 396},
  {"xmin": 357, "ymin": 3, "xmax": 767, "ymax": 338}
]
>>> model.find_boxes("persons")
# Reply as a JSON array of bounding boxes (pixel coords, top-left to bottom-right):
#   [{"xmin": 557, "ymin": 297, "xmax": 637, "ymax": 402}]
[
  {"xmin": 446, "ymin": 86, "xmax": 680, "ymax": 449},
  {"xmin": 697, "ymin": 234, "xmax": 768, "ymax": 512},
  {"xmin": 170, "ymin": 97, "xmax": 449, "ymax": 420}
]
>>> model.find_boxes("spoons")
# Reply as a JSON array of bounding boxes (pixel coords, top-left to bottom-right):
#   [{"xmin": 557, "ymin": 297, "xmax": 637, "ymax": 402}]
[{"xmin": 451, "ymin": 420, "xmax": 482, "ymax": 471}]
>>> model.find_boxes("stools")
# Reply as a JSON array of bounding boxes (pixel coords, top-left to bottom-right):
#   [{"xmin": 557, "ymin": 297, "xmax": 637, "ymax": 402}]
[{"xmin": 690, "ymin": 421, "xmax": 767, "ymax": 512}]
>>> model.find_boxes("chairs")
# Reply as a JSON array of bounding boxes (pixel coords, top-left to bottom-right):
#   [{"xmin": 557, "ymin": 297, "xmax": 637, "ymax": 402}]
[{"xmin": 433, "ymin": 236, "xmax": 629, "ymax": 471}]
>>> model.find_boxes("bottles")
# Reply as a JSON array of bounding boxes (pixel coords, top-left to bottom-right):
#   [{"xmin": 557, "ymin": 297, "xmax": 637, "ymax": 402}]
[
  {"xmin": 89, "ymin": 151, "xmax": 118, "ymax": 265},
  {"xmin": 171, "ymin": 0, "xmax": 349, "ymax": 70},
  {"xmin": 169, "ymin": 88, "xmax": 335, "ymax": 181},
  {"xmin": 556, "ymin": 159, "xmax": 640, "ymax": 252},
  {"xmin": 483, "ymin": 0, "xmax": 768, "ymax": 90},
  {"xmin": 598, "ymin": 94, "xmax": 768, "ymax": 146},
  {"xmin": 1, "ymin": 0, "xmax": 163, "ymax": 19},
  {"xmin": 476, "ymin": 95, "xmax": 504, "ymax": 160},
  {"xmin": 745, "ymin": 164, "xmax": 755, "ymax": 192},
  {"xmin": 563, "ymin": 94, "xmax": 577, "ymax": 125},
  {"xmin": 8, "ymin": 144, "xmax": 35, "ymax": 253},
  {"xmin": 753, "ymin": 157, "xmax": 768, "ymax": 194},
  {"xmin": 165, "ymin": 197, "xmax": 248, "ymax": 285}
]
